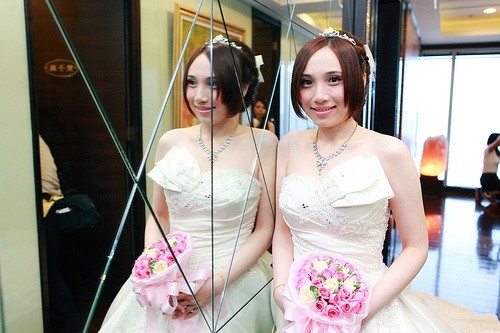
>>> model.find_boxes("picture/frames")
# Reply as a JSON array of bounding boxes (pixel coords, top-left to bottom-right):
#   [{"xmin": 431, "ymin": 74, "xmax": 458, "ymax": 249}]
[{"xmin": 171, "ymin": 4, "xmax": 246, "ymax": 131}]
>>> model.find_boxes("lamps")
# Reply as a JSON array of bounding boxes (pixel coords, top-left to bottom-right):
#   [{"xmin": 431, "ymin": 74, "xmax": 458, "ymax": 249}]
[{"xmin": 419, "ymin": 135, "xmax": 452, "ymax": 200}]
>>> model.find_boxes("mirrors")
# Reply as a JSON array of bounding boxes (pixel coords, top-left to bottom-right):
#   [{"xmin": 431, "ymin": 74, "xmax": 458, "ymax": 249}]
[{"xmin": 0, "ymin": 0, "xmax": 352, "ymax": 333}]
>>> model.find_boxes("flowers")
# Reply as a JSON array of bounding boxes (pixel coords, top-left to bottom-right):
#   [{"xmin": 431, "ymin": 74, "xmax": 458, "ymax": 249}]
[
  {"xmin": 131, "ymin": 232, "xmax": 207, "ymax": 309},
  {"xmin": 283, "ymin": 251, "xmax": 368, "ymax": 333}
]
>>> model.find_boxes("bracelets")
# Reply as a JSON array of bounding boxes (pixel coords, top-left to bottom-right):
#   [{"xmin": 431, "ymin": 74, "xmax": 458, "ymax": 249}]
[
  {"xmin": 217, "ymin": 278, "xmax": 227, "ymax": 295},
  {"xmin": 272, "ymin": 283, "xmax": 285, "ymax": 292}
]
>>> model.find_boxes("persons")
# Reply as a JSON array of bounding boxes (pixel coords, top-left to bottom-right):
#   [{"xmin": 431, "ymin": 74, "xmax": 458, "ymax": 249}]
[
  {"xmin": 101, "ymin": 34, "xmax": 278, "ymax": 333},
  {"xmin": 252, "ymin": 99, "xmax": 274, "ymax": 133},
  {"xmin": 475, "ymin": 133, "xmax": 500, "ymax": 207},
  {"xmin": 37, "ymin": 136, "xmax": 62, "ymax": 218},
  {"xmin": 272, "ymin": 29, "xmax": 500, "ymax": 333}
]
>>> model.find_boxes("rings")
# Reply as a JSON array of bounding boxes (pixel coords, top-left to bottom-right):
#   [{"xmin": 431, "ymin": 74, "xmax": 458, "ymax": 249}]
[{"xmin": 187, "ymin": 306, "xmax": 193, "ymax": 312}]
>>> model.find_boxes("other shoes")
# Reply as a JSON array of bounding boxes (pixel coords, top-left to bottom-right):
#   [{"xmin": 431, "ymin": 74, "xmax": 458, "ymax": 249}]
[{"xmin": 475, "ymin": 187, "xmax": 483, "ymax": 208}]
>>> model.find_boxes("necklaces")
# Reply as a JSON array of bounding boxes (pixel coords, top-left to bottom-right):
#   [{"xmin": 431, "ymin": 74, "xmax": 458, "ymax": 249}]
[
  {"xmin": 314, "ymin": 122, "xmax": 358, "ymax": 176},
  {"xmin": 198, "ymin": 120, "xmax": 241, "ymax": 161}
]
